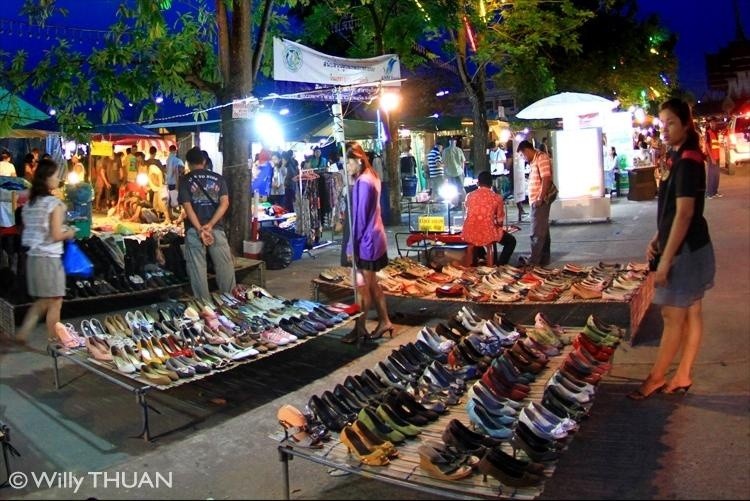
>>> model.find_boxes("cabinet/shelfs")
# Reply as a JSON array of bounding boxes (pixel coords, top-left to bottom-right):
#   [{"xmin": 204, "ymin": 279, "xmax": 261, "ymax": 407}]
[
  {"xmin": 311, "ymin": 256, "xmax": 655, "ymax": 344},
  {"xmin": 396, "ymin": 200, "xmax": 522, "ymax": 264},
  {"xmin": 0, "ymin": 258, "xmax": 269, "ymax": 341},
  {"xmin": 267, "ymin": 325, "xmax": 625, "ymax": 500},
  {"xmin": 46, "ymin": 283, "xmax": 367, "ymax": 442}
]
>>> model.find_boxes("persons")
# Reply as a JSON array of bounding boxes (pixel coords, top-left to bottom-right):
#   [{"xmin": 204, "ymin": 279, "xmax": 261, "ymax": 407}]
[{"xmin": 0, "ymin": 100, "xmax": 719, "ymax": 401}]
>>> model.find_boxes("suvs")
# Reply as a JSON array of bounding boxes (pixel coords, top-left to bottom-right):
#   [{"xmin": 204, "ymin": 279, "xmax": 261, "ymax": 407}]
[{"xmin": 692, "ymin": 113, "xmax": 750, "ymax": 175}]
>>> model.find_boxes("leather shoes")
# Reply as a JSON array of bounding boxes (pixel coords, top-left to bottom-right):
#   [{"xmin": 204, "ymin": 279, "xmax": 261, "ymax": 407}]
[
  {"xmin": 491, "ymin": 319, "xmax": 528, "ymax": 337},
  {"xmin": 194, "ymin": 349, "xmax": 227, "ymax": 369},
  {"xmin": 251, "ymin": 336, "xmax": 278, "ymax": 351},
  {"xmin": 236, "ymin": 335, "xmax": 267, "ymax": 353},
  {"xmin": 165, "ymin": 358, "xmax": 196, "ymax": 377},
  {"xmin": 149, "ymin": 361, "xmax": 179, "ymax": 382},
  {"xmin": 178, "ymin": 355, "xmax": 211, "ymax": 373},
  {"xmin": 270, "ymin": 326, "xmax": 299, "ymax": 342},
  {"xmin": 65, "ymin": 268, "xmax": 182, "ymax": 299},
  {"xmin": 228, "ymin": 340, "xmax": 261, "ymax": 358},
  {"xmin": 202, "ymin": 346, "xmax": 235, "ymax": 366},
  {"xmin": 492, "ymin": 312, "xmax": 527, "ymax": 329},
  {"xmin": 317, "ymin": 261, "xmax": 353, "ymax": 288},
  {"xmin": 261, "ymin": 329, "xmax": 290, "ymax": 347},
  {"xmin": 381, "ymin": 257, "xmax": 648, "ymax": 304},
  {"xmin": 139, "ymin": 364, "xmax": 171, "ymax": 384},
  {"xmin": 213, "ymin": 284, "xmax": 364, "ymax": 343},
  {"xmin": 218, "ymin": 343, "xmax": 251, "ymax": 362},
  {"xmin": 126, "ymin": 296, "xmax": 213, "ymax": 347}
]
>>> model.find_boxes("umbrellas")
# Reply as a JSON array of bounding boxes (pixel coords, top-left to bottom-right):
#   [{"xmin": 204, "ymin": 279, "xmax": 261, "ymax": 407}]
[{"xmin": 515, "ymin": 92, "xmax": 618, "ymax": 127}]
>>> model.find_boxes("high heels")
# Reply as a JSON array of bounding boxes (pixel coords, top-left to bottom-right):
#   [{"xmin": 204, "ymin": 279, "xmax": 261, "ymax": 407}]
[
  {"xmin": 546, "ymin": 373, "xmax": 590, "ymax": 404},
  {"xmin": 586, "ymin": 314, "xmax": 626, "ymax": 338},
  {"xmin": 535, "ymin": 320, "xmax": 575, "ymax": 345},
  {"xmin": 487, "ymin": 367, "xmax": 532, "ymax": 391},
  {"xmin": 541, "ymin": 394, "xmax": 586, "ymax": 423},
  {"xmin": 351, "ymin": 420, "xmax": 400, "ymax": 459},
  {"xmin": 122, "ymin": 345, "xmax": 146, "ymax": 371},
  {"xmin": 553, "ymin": 368, "xmax": 596, "ymax": 395},
  {"xmin": 528, "ymin": 399, "xmax": 579, "ymax": 433},
  {"xmin": 461, "ymin": 305, "xmax": 490, "ymax": 325},
  {"xmin": 110, "ymin": 346, "xmax": 137, "ymax": 374},
  {"xmin": 390, "ymin": 388, "xmax": 439, "ymax": 421},
  {"xmin": 533, "ymin": 312, "xmax": 565, "ymax": 331},
  {"xmin": 519, "ymin": 407, "xmax": 568, "ymax": 440},
  {"xmin": 457, "ymin": 311, "xmax": 485, "ymax": 334},
  {"xmin": 376, "ymin": 404, "xmax": 423, "ymax": 437},
  {"xmin": 483, "ymin": 445, "xmax": 543, "ymax": 475},
  {"xmin": 383, "ymin": 394, "xmax": 431, "ymax": 426},
  {"xmin": 340, "ymin": 327, "xmax": 369, "ymax": 344},
  {"xmin": 157, "ymin": 335, "xmax": 178, "ymax": 357},
  {"xmin": 418, "ymin": 445, "xmax": 473, "ymax": 482},
  {"xmin": 406, "ymin": 381, "xmax": 451, "ymax": 413},
  {"xmin": 354, "ymin": 372, "xmax": 386, "ymax": 399},
  {"xmin": 423, "ymin": 327, "xmax": 455, "ymax": 346},
  {"xmin": 420, "ymin": 375, "xmax": 458, "ymax": 406},
  {"xmin": 136, "ymin": 337, "xmax": 164, "ymax": 365},
  {"xmin": 546, "ymin": 386, "xmax": 591, "ymax": 419},
  {"xmin": 482, "ymin": 321, "xmax": 513, "ymax": 346},
  {"xmin": 468, "ymin": 384, "xmax": 519, "ymax": 416},
  {"xmin": 473, "ymin": 379, "xmax": 523, "ymax": 410},
  {"xmin": 360, "ymin": 368, "xmax": 394, "ymax": 393},
  {"xmin": 443, "ymin": 426, "xmax": 487, "ymax": 458},
  {"xmin": 367, "ymin": 322, "xmax": 393, "ymax": 341},
  {"xmin": 479, "ymin": 454, "xmax": 542, "ymax": 488},
  {"xmin": 445, "ymin": 418, "xmax": 496, "ymax": 446},
  {"xmin": 169, "ymin": 333, "xmax": 191, "ymax": 356},
  {"xmin": 482, "ymin": 372, "xmax": 529, "ymax": 400},
  {"xmin": 583, "ymin": 325, "xmax": 621, "ymax": 346},
  {"xmin": 436, "ymin": 320, "xmax": 460, "ymax": 341},
  {"xmin": 465, "ymin": 398, "xmax": 516, "ymax": 426},
  {"xmin": 321, "ymin": 390, "xmax": 357, "ymax": 423},
  {"xmin": 422, "ymin": 438, "xmax": 481, "ymax": 470},
  {"xmin": 446, "ymin": 315, "xmax": 471, "ymax": 336},
  {"xmin": 148, "ymin": 335, "xmax": 171, "ymax": 362},
  {"xmin": 661, "ymin": 382, "xmax": 693, "ymax": 397},
  {"xmin": 628, "ymin": 382, "xmax": 666, "ymax": 401},
  {"xmin": 334, "ymin": 384, "xmax": 365, "ymax": 412},
  {"xmin": 345, "ymin": 375, "xmax": 375, "ymax": 405},
  {"xmin": 340, "ymin": 426, "xmax": 390, "ymax": 467},
  {"xmin": 469, "ymin": 405, "xmax": 513, "ymax": 441},
  {"xmin": 373, "ymin": 332, "xmax": 624, "ymax": 384},
  {"xmin": 488, "ymin": 317, "xmax": 521, "ymax": 340},
  {"xmin": 297, "ymin": 407, "xmax": 333, "ymax": 442},
  {"xmin": 308, "ymin": 394, "xmax": 352, "ymax": 432},
  {"xmin": 279, "ymin": 418, "xmax": 325, "ymax": 449},
  {"xmin": 55, "ymin": 312, "xmax": 131, "ymax": 362},
  {"xmin": 415, "ymin": 329, "xmax": 446, "ymax": 352},
  {"xmin": 510, "ymin": 429, "xmax": 560, "ymax": 462},
  {"xmin": 357, "ymin": 408, "xmax": 406, "ymax": 445},
  {"xmin": 512, "ymin": 419, "xmax": 565, "ymax": 452}
]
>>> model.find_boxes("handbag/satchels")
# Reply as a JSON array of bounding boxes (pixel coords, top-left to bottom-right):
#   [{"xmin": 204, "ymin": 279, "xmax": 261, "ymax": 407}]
[
  {"xmin": 649, "ymin": 250, "xmax": 663, "ymax": 272},
  {"xmin": 544, "ymin": 184, "xmax": 560, "ymax": 206}
]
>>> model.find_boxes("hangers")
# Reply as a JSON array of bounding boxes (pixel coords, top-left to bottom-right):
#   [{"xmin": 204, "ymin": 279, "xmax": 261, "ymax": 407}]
[{"xmin": 292, "ymin": 169, "xmax": 320, "ymax": 181}]
